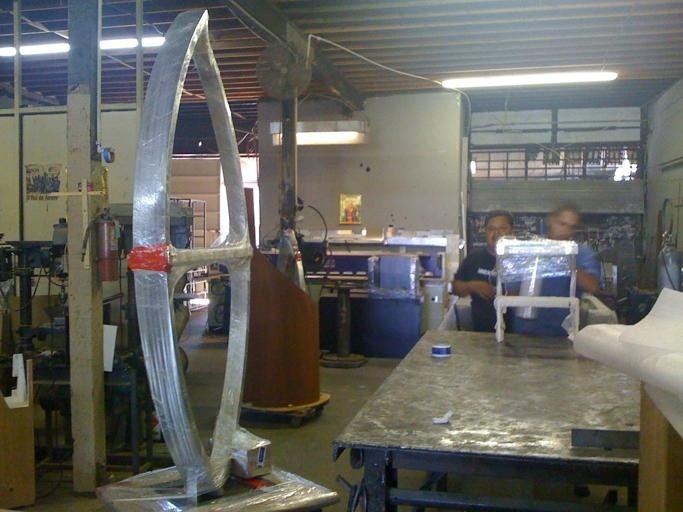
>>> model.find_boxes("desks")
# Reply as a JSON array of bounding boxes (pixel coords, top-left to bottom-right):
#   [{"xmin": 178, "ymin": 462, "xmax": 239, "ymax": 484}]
[
  {"xmin": 331, "ymin": 328, "xmax": 641, "ymax": 512},
  {"xmin": 636, "ymin": 379, "xmax": 683, "ymax": 511}
]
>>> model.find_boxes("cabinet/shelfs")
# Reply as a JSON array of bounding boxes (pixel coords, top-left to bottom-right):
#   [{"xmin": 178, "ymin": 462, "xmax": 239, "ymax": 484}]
[
  {"xmin": 170, "ymin": 198, "xmax": 210, "ymax": 315},
  {"xmin": 260, "ymin": 234, "xmax": 466, "ymax": 360}
]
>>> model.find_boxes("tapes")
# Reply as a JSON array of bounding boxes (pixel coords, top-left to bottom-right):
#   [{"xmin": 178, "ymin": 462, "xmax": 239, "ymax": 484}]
[
  {"xmin": 103, "ymin": 147, "xmax": 115, "ymax": 163},
  {"xmin": 432, "ymin": 344, "xmax": 452, "ymax": 357}
]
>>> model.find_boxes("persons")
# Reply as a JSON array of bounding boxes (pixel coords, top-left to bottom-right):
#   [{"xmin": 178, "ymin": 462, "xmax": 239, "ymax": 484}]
[
  {"xmin": 509, "ymin": 205, "xmax": 602, "ymax": 337},
  {"xmin": 452, "ymin": 210, "xmax": 514, "ymax": 332}
]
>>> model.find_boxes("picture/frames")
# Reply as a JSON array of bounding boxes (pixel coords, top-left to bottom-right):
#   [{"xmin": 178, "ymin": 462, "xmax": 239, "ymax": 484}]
[{"xmin": 337, "ymin": 193, "xmax": 363, "ymax": 225}]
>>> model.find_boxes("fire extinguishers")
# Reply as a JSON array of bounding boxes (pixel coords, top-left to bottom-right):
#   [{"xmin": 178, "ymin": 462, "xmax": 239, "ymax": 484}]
[
  {"xmin": 95, "ymin": 207, "xmax": 119, "ymax": 282},
  {"xmin": 657, "ymin": 231, "xmax": 681, "ymax": 291}
]
